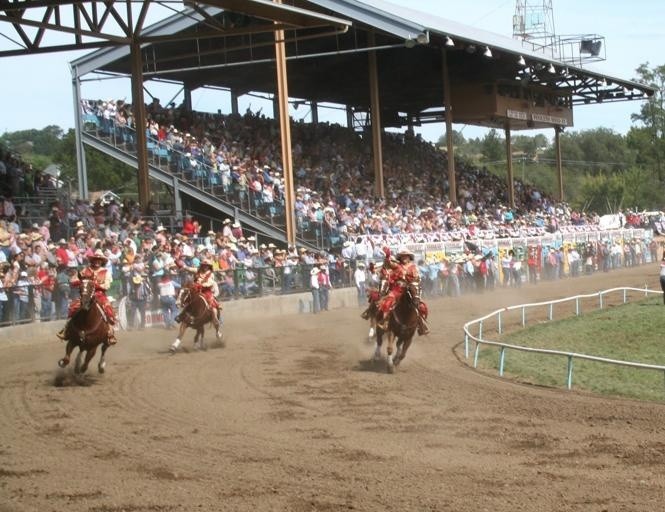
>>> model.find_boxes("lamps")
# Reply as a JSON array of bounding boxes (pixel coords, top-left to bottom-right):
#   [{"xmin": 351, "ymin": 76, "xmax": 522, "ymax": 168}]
[{"xmin": 445, "ymin": 35, "xmax": 557, "ymax": 74}]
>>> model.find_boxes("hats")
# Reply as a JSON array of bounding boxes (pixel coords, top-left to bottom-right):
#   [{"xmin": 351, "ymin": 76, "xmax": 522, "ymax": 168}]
[{"xmin": 0, "ymin": 97, "xmax": 665, "ymax": 285}]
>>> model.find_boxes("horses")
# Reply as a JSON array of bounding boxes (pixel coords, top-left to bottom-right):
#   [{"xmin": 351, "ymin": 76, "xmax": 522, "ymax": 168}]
[
  {"xmin": 169, "ymin": 281, "xmax": 222, "ymax": 353},
  {"xmin": 367, "ymin": 268, "xmax": 419, "ymax": 375},
  {"xmin": 58, "ymin": 270, "xmax": 110, "ymax": 385}
]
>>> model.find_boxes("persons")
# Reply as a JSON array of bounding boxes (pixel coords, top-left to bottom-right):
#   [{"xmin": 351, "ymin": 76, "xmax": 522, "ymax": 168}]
[{"xmin": 48, "ymin": 248, "xmax": 119, "ymax": 345}]
